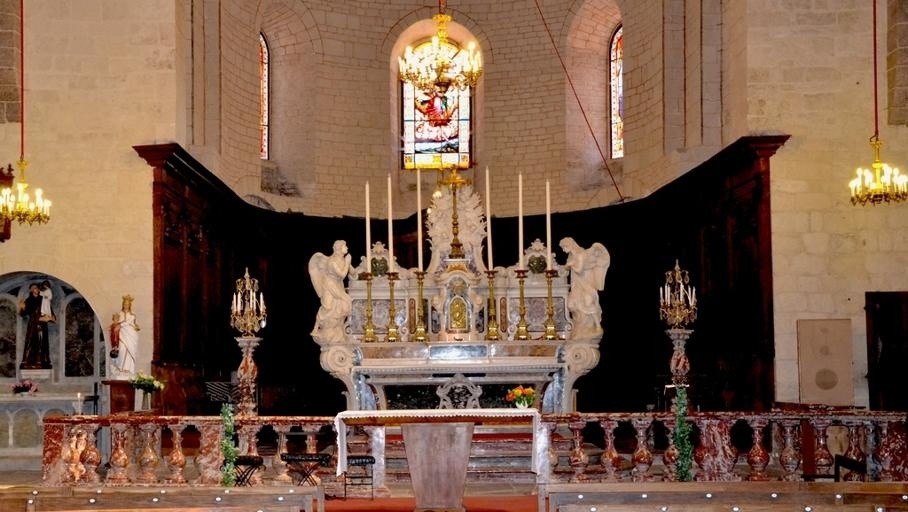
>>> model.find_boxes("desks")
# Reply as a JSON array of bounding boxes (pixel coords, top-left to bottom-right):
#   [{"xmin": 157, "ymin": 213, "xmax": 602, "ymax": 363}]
[{"xmin": 221, "ymin": 456, "xmax": 375, "ymax": 500}]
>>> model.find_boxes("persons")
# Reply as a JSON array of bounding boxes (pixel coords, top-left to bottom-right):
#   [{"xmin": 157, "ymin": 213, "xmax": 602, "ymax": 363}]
[
  {"xmin": 559, "ymin": 236, "xmax": 602, "ymax": 336},
  {"xmin": 104, "ymin": 312, "xmax": 126, "ymax": 351},
  {"xmin": 311, "ymin": 240, "xmax": 360, "ymax": 336},
  {"xmin": 19, "ymin": 284, "xmax": 57, "ymax": 369},
  {"xmin": 34, "ymin": 281, "xmax": 52, "ymax": 317},
  {"xmin": 108, "ymin": 294, "xmax": 139, "ymax": 380}
]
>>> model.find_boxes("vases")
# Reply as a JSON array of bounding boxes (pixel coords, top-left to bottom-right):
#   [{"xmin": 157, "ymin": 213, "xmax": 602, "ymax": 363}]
[
  {"xmin": 142, "ymin": 392, "xmax": 148, "ymax": 410},
  {"xmin": 515, "ymin": 400, "xmax": 529, "ymax": 408}
]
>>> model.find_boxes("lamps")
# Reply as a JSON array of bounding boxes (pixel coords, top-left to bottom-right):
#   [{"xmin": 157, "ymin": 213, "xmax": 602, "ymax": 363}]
[
  {"xmin": 850, "ymin": 0, "xmax": 908, "ymax": 207},
  {"xmin": 399, "ymin": 0, "xmax": 483, "ymax": 99},
  {"xmin": 0, "ymin": 0, "xmax": 50, "ymax": 227}
]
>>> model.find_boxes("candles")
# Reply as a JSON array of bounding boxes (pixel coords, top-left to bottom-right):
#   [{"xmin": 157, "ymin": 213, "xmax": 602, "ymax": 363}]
[
  {"xmin": 231, "ymin": 289, "xmax": 265, "ymax": 314},
  {"xmin": 660, "ymin": 283, "xmax": 696, "ymax": 306},
  {"xmin": 365, "ymin": 166, "xmax": 552, "ymax": 274}
]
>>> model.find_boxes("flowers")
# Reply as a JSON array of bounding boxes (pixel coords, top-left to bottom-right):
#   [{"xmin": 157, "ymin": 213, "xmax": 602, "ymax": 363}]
[
  {"xmin": 128, "ymin": 370, "xmax": 164, "ymax": 394},
  {"xmin": 11, "ymin": 379, "xmax": 37, "ymax": 396},
  {"xmin": 506, "ymin": 385, "xmax": 537, "ymax": 406}
]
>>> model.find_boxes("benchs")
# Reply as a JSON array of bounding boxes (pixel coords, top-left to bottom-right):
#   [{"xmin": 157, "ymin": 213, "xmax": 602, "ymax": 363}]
[
  {"xmin": 538, "ymin": 482, "xmax": 908, "ymax": 512},
  {"xmin": 0, "ymin": 486, "xmax": 324, "ymax": 512}
]
